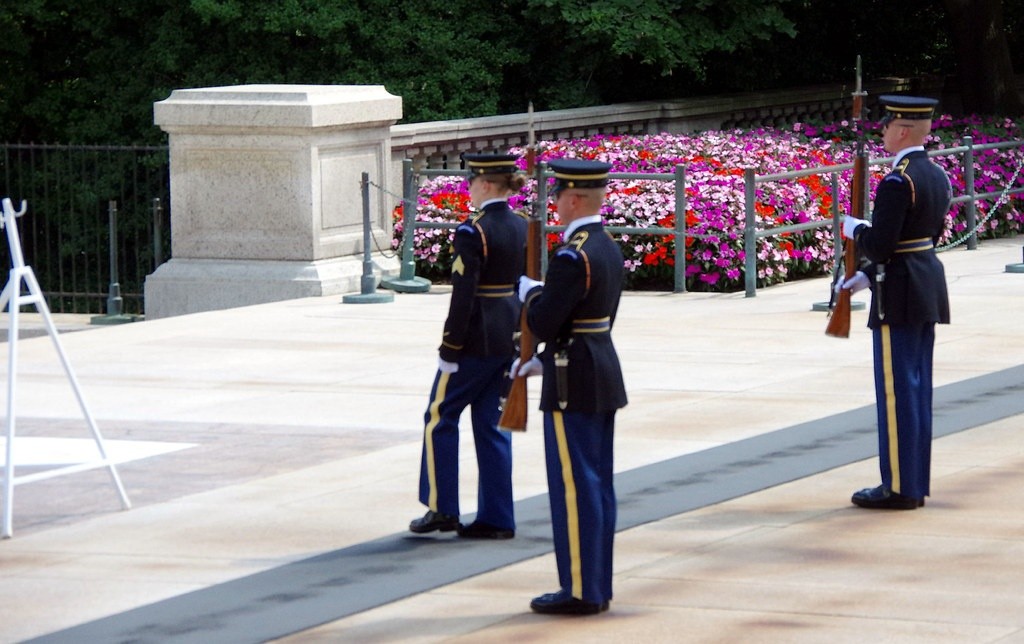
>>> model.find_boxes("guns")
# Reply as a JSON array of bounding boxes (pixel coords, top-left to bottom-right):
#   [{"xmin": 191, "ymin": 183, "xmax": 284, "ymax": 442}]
[
  {"xmin": 496, "ymin": 101, "xmax": 543, "ymax": 433},
  {"xmin": 824, "ymin": 55, "xmax": 870, "ymax": 339}
]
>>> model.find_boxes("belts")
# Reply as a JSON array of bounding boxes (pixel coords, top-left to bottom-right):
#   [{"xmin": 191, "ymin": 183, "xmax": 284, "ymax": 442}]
[
  {"xmin": 897, "ymin": 237, "xmax": 936, "ymax": 253},
  {"xmin": 570, "ymin": 317, "xmax": 612, "ymax": 333},
  {"xmin": 474, "ymin": 284, "xmax": 514, "ymax": 297}
]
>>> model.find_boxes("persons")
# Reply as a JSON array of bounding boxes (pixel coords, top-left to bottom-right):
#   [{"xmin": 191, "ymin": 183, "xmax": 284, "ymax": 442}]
[
  {"xmin": 410, "ymin": 154, "xmax": 527, "ymax": 540},
  {"xmin": 835, "ymin": 97, "xmax": 951, "ymax": 510},
  {"xmin": 510, "ymin": 159, "xmax": 628, "ymax": 617}
]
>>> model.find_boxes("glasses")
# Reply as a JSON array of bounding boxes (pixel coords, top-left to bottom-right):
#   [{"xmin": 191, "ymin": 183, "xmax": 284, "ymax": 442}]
[
  {"xmin": 555, "ymin": 191, "xmax": 561, "ymax": 202},
  {"xmin": 469, "ymin": 179, "xmax": 476, "ymax": 186},
  {"xmin": 885, "ymin": 123, "xmax": 914, "ymax": 129}
]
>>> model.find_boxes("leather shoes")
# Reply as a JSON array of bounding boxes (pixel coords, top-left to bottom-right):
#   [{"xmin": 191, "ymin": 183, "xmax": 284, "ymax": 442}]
[
  {"xmin": 851, "ymin": 487, "xmax": 925, "ymax": 510},
  {"xmin": 409, "ymin": 510, "xmax": 459, "ymax": 534},
  {"xmin": 457, "ymin": 519, "xmax": 516, "ymax": 539},
  {"xmin": 542, "ymin": 590, "xmax": 609, "ymax": 612},
  {"xmin": 530, "ymin": 598, "xmax": 601, "ymax": 615}
]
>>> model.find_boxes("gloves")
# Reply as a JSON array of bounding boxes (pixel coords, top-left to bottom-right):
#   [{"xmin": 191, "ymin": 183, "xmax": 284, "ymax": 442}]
[
  {"xmin": 509, "ymin": 356, "xmax": 543, "ymax": 380},
  {"xmin": 843, "ymin": 215, "xmax": 870, "ymax": 241},
  {"xmin": 518, "ymin": 275, "xmax": 545, "ymax": 303},
  {"xmin": 437, "ymin": 355, "xmax": 459, "ymax": 374},
  {"xmin": 833, "ymin": 271, "xmax": 871, "ymax": 297}
]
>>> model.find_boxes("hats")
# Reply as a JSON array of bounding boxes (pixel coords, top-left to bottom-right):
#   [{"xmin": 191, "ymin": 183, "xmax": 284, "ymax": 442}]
[
  {"xmin": 877, "ymin": 94, "xmax": 940, "ymax": 125},
  {"xmin": 460, "ymin": 153, "xmax": 519, "ymax": 181},
  {"xmin": 547, "ymin": 161, "xmax": 613, "ymax": 195}
]
